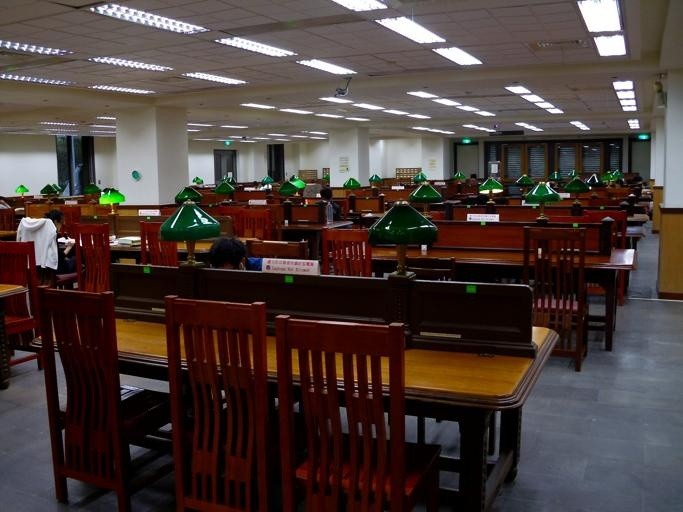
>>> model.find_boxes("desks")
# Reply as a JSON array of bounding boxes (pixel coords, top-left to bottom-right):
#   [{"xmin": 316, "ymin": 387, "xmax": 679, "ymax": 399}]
[{"xmin": 27, "ymin": 314, "xmax": 563, "ymax": 512}]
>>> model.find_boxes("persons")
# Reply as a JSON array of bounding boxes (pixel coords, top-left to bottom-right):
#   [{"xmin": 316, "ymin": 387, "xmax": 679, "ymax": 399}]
[
  {"xmin": 0, "ymin": 196, "xmax": 11, "ymax": 209},
  {"xmin": 44, "ymin": 210, "xmax": 78, "ymax": 273},
  {"xmin": 319, "ymin": 187, "xmax": 341, "ymax": 219},
  {"xmin": 208, "ymin": 238, "xmax": 249, "ymax": 270}
]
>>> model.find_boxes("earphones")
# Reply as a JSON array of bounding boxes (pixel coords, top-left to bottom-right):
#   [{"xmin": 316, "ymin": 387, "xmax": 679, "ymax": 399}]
[{"xmin": 240, "ymin": 262, "xmax": 244, "ymax": 268}]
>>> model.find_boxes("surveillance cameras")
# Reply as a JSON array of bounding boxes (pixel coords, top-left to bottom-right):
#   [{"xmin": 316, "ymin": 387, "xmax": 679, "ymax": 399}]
[{"xmin": 334, "ymin": 87, "xmax": 349, "ymax": 97}]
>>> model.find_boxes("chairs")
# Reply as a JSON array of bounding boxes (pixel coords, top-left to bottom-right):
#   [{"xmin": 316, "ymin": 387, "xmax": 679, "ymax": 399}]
[
  {"xmin": 162, "ymin": 291, "xmax": 285, "ymax": 512},
  {"xmin": 275, "ymin": 315, "xmax": 445, "ymax": 512},
  {"xmin": 0, "ymin": 175, "xmax": 657, "ymax": 378},
  {"xmin": 31, "ymin": 281, "xmax": 172, "ymax": 512}
]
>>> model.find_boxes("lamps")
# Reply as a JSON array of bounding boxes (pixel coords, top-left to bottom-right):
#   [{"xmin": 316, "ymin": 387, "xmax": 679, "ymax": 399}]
[{"xmin": 6, "ymin": 169, "xmax": 622, "ymax": 278}]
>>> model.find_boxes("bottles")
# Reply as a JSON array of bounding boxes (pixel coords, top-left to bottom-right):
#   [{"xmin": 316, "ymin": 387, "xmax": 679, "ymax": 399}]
[{"xmin": 325, "ymin": 201, "xmax": 333, "ymax": 225}]
